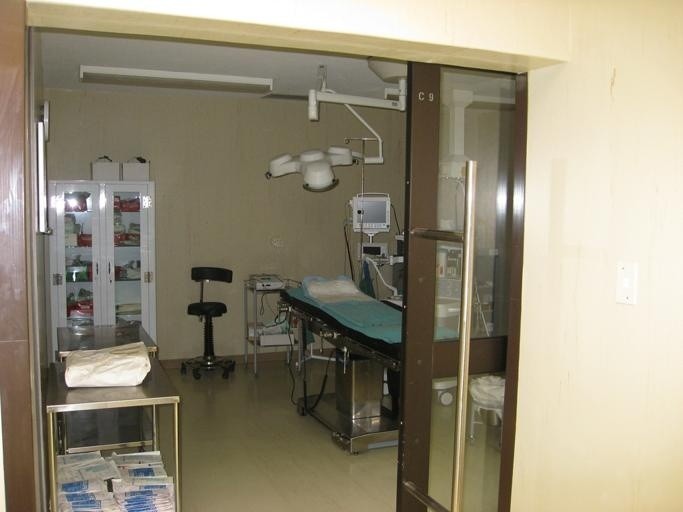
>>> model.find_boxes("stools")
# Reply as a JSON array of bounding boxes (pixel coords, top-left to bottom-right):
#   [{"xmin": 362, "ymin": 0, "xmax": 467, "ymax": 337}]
[{"xmin": 468, "ymin": 375, "xmax": 506, "ymax": 445}]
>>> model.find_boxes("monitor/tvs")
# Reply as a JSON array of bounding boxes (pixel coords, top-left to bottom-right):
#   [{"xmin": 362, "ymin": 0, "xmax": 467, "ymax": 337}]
[{"xmin": 353, "ymin": 197, "xmax": 391, "ymax": 237}]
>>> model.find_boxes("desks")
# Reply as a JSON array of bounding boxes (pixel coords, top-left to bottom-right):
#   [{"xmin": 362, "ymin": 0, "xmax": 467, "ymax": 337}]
[{"xmin": 43, "ymin": 322, "xmax": 181, "ymax": 512}]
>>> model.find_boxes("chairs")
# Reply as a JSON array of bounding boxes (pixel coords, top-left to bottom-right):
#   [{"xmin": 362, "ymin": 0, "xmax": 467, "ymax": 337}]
[{"xmin": 179, "ymin": 264, "xmax": 236, "ymax": 381}]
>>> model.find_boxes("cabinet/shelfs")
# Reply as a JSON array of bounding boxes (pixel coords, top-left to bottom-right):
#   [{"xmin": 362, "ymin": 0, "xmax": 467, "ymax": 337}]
[{"xmin": 46, "ymin": 178, "xmax": 159, "ymax": 371}]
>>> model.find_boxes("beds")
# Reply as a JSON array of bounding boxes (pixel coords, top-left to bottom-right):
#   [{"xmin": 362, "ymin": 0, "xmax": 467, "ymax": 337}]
[{"xmin": 278, "ymin": 272, "xmax": 459, "ymax": 454}]
